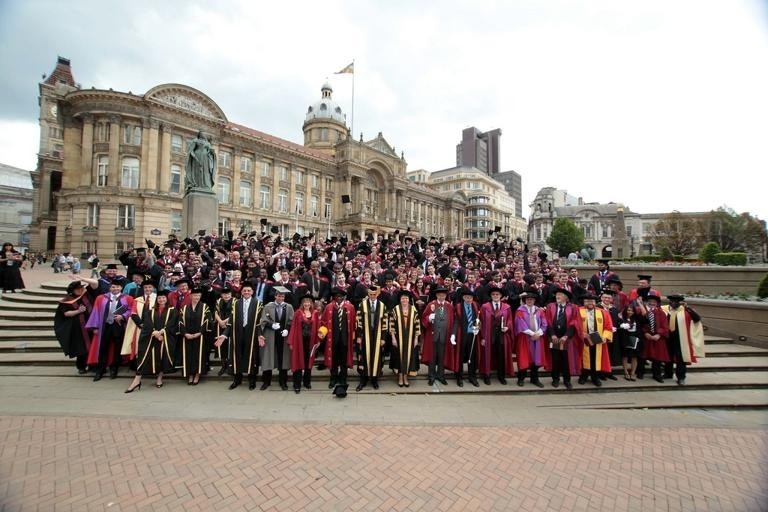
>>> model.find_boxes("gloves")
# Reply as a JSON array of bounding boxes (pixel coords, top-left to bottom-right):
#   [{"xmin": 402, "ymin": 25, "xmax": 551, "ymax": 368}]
[
  {"xmin": 281, "ymin": 330, "xmax": 288, "ymax": 337},
  {"xmin": 612, "ymin": 327, "xmax": 617, "ymax": 332},
  {"xmin": 620, "ymin": 323, "xmax": 630, "ymax": 330},
  {"xmin": 450, "ymin": 335, "xmax": 456, "ymax": 345}
]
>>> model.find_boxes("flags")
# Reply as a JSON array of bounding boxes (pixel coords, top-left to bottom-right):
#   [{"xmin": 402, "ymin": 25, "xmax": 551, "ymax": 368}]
[{"xmin": 333, "ymin": 62, "xmax": 354, "ymax": 74}]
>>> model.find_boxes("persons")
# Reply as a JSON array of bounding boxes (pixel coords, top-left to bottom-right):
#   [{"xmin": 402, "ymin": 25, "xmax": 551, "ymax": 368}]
[
  {"xmin": 183, "ymin": 130, "xmax": 217, "ymax": 187},
  {"xmin": 23, "ymin": 251, "xmax": 47, "ymax": 269},
  {"xmin": 51, "ymin": 228, "xmax": 702, "ymax": 398},
  {"xmin": 0, "ymin": 242, "xmax": 25, "ymax": 293}
]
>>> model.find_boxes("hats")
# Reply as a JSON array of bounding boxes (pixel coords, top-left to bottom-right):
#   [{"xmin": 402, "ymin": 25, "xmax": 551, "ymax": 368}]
[{"xmin": 332, "ymin": 383, "xmax": 349, "ymax": 398}]
